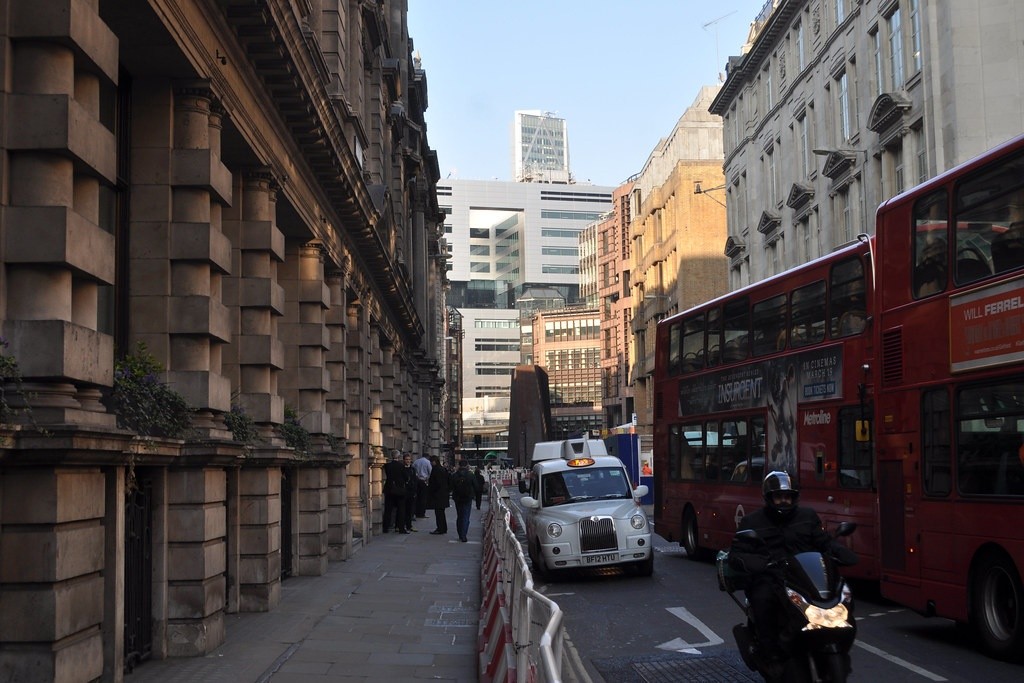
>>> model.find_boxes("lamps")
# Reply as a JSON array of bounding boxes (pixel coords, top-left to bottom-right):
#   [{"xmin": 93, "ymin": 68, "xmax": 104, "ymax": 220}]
[
  {"xmin": 644, "ymin": 294, "xmax": 673, "ymax": 303},
  {"xmin": 693, "ymin": 180, "xmax": 727, "ymax": 208},
  {"xmin": 812, "ymin": 147, "xmax": 868, "ymax": 157},
  {"xmin": 216, "ymin": 49, "xmax": 227, "ymax": 66},
  {"xmin": 319, "ymin": 214, "xmax": 327, "ymax": 224}
]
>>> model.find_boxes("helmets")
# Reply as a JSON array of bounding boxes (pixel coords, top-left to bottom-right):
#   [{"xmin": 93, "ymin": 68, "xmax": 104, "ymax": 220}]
[{"xmin": 760, "ymin": 470, "xmax": 801, "ymax": 520}]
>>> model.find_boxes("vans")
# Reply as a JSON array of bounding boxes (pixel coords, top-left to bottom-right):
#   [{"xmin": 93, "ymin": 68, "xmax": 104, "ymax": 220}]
[
  {"xmin": 677, "ymin": 430, "xmax": 734, "ymax": 480},
  {"xmin": 517, "ymin": 439, "xmax": 655, "ymax": 583}
]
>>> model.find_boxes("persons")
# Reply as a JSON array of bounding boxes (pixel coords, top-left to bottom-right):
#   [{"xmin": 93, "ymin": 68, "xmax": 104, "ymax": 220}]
[
  {"xmin": 382, "ymin": 450, "xmax": 410, "ymax": 534},
  {"xmin": 395, "ymin": 453, "xmax": 419, "ymax": 532},
  {"xmin": 412, "ymin": 453, "xmax": 433, "ymax": 518},
  {"xmin": 841, "ymin": 281, "xmax": 866, "ymax": 333},
  {"xmin": 918, "ymin": 237, "xmax": 947, "ymax": 297},
  {"xmin": 426, "ymin": 455, "xmax": 449, "ymax": 534},
  {"xmin": 442, "ymin": 462, "xmax": 446, "ymax": 468},
  {"xmin": 729, "ymin": 470, "xmax": 858, "ymax": 678},
  {"xmin": 446, "ymin": 460, "xmax": 479, "ymax": 542},
  {"xmin": 473, "ymin": 469, "xmax": 485, "ymax": 511}
]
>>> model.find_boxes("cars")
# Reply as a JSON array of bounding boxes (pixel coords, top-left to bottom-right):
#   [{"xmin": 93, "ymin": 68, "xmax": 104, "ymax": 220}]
[{"xmin": 729, "ymin": 457, "xmax": 872, "ymax": 490}]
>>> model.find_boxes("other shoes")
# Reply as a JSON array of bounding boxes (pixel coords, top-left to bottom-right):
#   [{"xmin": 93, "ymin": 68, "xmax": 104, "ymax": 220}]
[
  {"xmin": 430, "ymin": 530, "xmax": 444, "ymax": 535},
  {"xmin": 459, "ymin": 535, "xmax": 462, "ymax": 539},
  {"xmin": 395, "ymin": 529, "xmax": 400, "ymax": 531},
  {"xmin": 398, "ymin": 528, "xmax": 411, "ymax": 535},
  {"xmin": 462, "ymin": 535, "xmax": 468, "ymax": 542},
  {"xmin": 407, "ymin": 527, "xmax": 418, "ymax": 533}
]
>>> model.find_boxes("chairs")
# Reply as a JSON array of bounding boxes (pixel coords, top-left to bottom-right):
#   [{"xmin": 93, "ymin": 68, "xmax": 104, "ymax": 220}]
[
  {"xmin": 838, "ymin": 310, "xmax": 866, "ymax": 337},
  {"xmin": 683, "ymin": 349, "xmax": 704, "ymax": 373},
  {"xmin": 754, "ymin": 328, "xmax": 787, "ymax": 355},
  {"xmin": 724, "ymin": 334, "xmax": 748, "ymax": 364},
  {"xmin": 670, "ymin": 355, "xmax": 680, "ymax": 376},
  {"xmin": 917, "ymin": 224, "xmax": 1024, "ymax": 300},
  {"xmin": 708, "ymin": 345, "xmax": 720, "ymax": 368}
]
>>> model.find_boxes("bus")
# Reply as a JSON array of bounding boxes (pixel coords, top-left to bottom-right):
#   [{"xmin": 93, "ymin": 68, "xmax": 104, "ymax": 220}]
[
  {"xmin": 868, "ymin": 137, "xmax": 1024, "ymax": 661},
  {"xmin": 650, "ymin": 232, "xmax": 881, "ymax": 590}
]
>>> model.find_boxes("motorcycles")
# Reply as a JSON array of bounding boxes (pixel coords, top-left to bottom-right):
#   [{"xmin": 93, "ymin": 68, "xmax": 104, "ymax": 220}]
[{"xmin": 716, "ymin": 522, "xmax": 879, "ymax": 683}]
